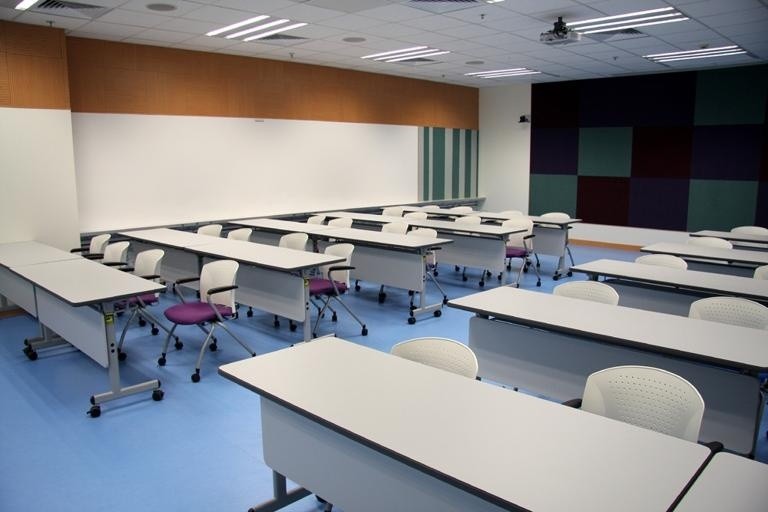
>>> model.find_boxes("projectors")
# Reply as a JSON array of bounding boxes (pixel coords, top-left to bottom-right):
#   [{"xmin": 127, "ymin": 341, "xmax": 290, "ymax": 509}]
[{"xmin": 540, "ymin": 32, "xmax": 578, "ymax": 45}]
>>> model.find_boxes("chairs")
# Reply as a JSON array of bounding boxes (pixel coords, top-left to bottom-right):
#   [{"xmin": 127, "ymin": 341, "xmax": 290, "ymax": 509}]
[{"xmin": 0, "ymin": 204, "xmax": 768, "ymax": 512}]
